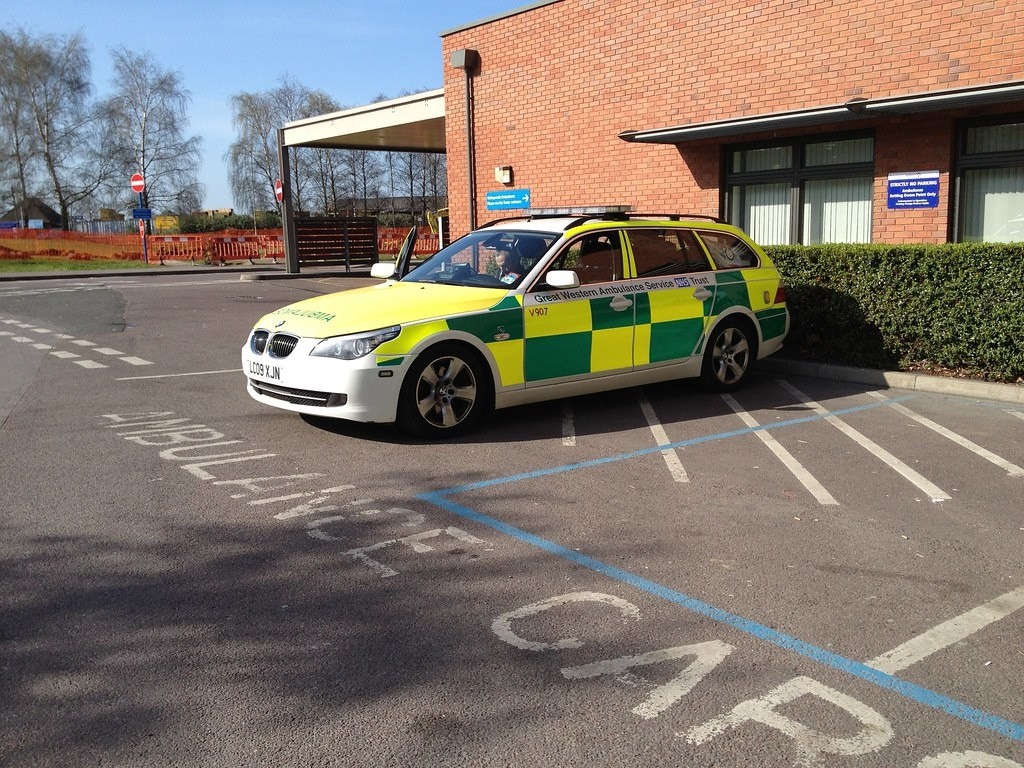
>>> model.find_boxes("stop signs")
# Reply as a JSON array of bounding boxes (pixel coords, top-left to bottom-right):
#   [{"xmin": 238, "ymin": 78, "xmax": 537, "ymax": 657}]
[
  {"xmin": 130, "ymin": 174, "xmax": 144, "ymax": 193},
  {"xmin": 138, "ymin": 219, "xmax": 146, "ymax": 238},
  {"xmin": 275, "ymin": 179, "xmax": 284, "ymax": 203}
]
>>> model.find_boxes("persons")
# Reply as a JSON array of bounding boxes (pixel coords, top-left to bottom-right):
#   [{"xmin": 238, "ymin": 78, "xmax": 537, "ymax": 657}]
[{"xmin": 492, "ymin": 249, "xmax": 526, "ymax": 280}]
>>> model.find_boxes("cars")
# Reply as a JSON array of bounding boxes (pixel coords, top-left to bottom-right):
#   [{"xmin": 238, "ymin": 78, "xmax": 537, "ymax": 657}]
[{"xmin": 239, "ymin": 212, "xmax": 792, "ymax": 438}]
